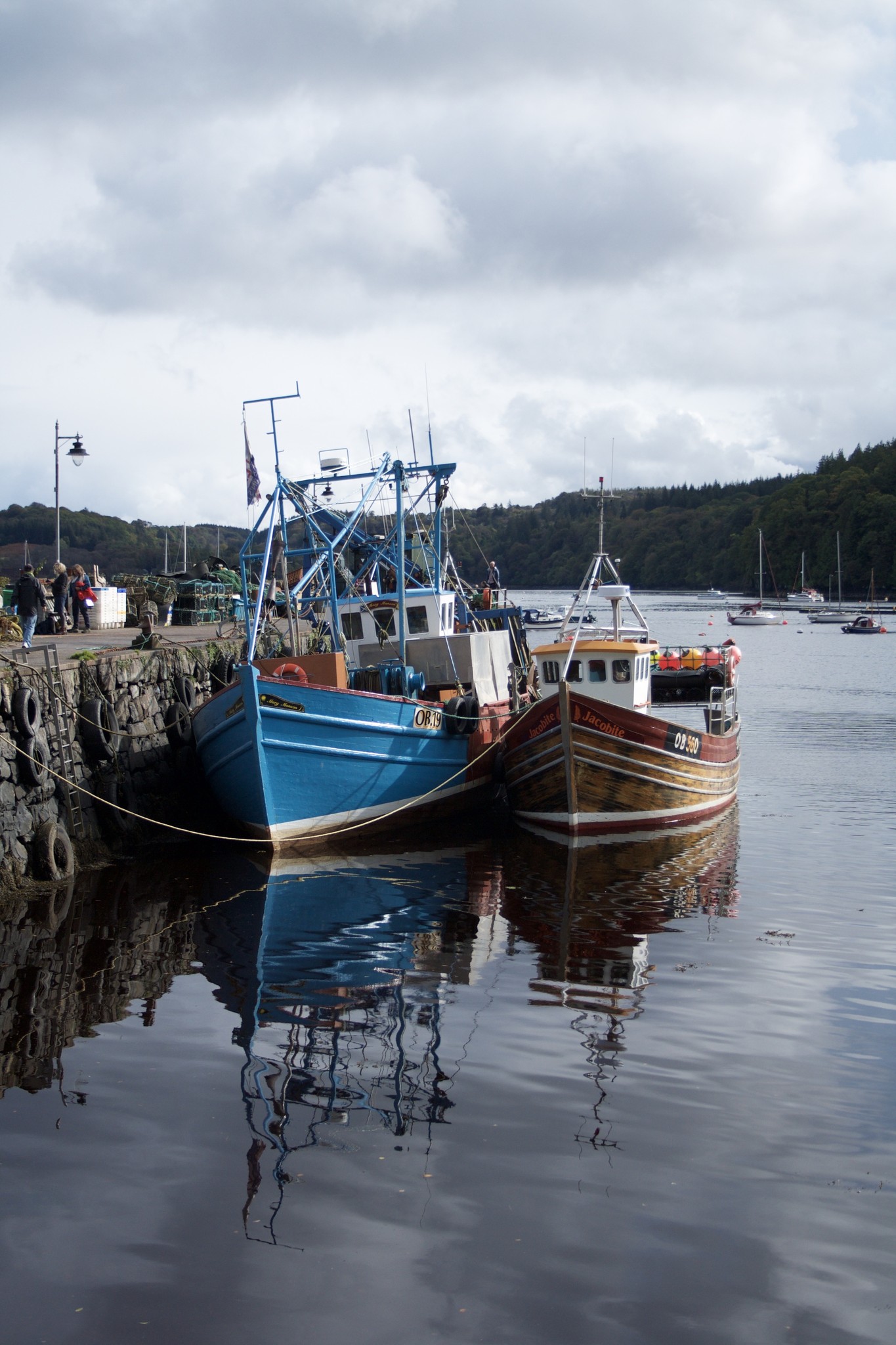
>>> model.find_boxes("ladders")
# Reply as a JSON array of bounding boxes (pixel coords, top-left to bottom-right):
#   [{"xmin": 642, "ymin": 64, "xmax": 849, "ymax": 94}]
[{"xmin": 12, "ymin": 644, "xmax": 84, "ymax": 836}]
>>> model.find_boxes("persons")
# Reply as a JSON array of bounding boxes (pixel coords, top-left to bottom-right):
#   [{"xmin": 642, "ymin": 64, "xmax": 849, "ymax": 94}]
[
  {"xmin": 475, "ymin": 561, "xmax": 500, "ymax": 600},
  {"xmin": 67, "ymin": 564, "xmax": 91, "ymax": 633},
  {"xmin": 10, "ymin": 564, "xmax": 47, "ymax": 654},
  {"xmin": 46, "ymin": 562, "xmax": 70, "ymax": 635}
]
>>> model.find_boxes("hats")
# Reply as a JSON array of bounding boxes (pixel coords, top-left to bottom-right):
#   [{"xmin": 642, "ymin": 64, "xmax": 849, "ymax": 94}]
[{"xmin": 24, "ymin": 563, "xmax": 34, "ymax": 571}]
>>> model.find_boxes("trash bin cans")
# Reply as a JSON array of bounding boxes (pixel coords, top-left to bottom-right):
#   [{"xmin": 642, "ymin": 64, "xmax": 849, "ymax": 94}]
[
  {"xmin": 231, "ymin": 598, "xmax": 250, "ymax": 621},
  {"xmin": 2, "ymin": 589, "xmax": 13, "ymax": 606}
]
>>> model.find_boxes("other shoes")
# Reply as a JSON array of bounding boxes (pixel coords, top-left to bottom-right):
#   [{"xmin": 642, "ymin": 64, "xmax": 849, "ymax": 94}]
[
  {"xmin": 67, "ymin": 626, "xmax": 79, "ymax": 633},
  {"xmin": 54, "ymin": 628, "xmax": 67, "ymax": 635},
  {"xmin": 81, "ymin": 628, "xmax": 91, "ymax": 633},
  {"xmin": 22, "ymin": 642, "xmax": 30, "ymax": 654}
]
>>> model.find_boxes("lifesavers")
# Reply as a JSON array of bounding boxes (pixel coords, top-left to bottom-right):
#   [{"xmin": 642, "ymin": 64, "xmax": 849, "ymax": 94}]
[
  {"xmin": 465, "ymin": 697, "xmax": 480, "ymax": 731},
  {"xmin": 37, "ymin": 879, "xmax": 73, "ymax": 932},
  {"xmin": 483, "ymin": 587, "xmax": 492, "ymax": 608},
  {"xmin": 84, "ymin": 695, "xmax": 139, "ymax": 836},
  {"xmin": 33, "ymin": 822, "xmax": 75, "ymax": 880},
  {"xmin": 12, "ymin": 686, "xmax": 49, "ymax": 788},
  {"xmin": 445, "ymin": 697, "xmax": 467, "ymax": 733},
  {"xmin": 270, "ymin": 664, "xmax": 308, "ymax": 685},
  {"xmin": 174, "ymin": 676, "xmax": 198, "ymax": 711},
  {"xmin": 217, "ymin": 654, "xmax": 236, "ymax": 689},
  {"xmin": 168, "ymin": 701, "xmax": 192, "ymax": 746}
]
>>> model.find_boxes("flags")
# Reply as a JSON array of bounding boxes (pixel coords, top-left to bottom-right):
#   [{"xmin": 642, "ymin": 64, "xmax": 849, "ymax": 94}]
[{"xmin": 240, "ymin": 410, "xmax": 262, "ymax": 512}]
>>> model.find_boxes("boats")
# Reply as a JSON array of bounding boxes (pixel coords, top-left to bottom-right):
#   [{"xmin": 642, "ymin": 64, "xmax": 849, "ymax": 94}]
[
  {"xmin": 493, "ymin": 472, "xmax": 744, "ymax": 853},
  {"xmin": 696, "ymin": 588, "xmax": 728, "ymax": 600},
  {"xmin": 186, "ymin": 377, "xmax": 540, "ymax": 853},
  {"xmin": 521, "ymin": 608, "xmax": 565, "ymax": 629},
  {"xmin": 840, "ymin": 567, "xmax": 884, "ymax": 633},
  {"xmin": 555, "ymin": 605, "xmax": 598, "ymax": 624}
]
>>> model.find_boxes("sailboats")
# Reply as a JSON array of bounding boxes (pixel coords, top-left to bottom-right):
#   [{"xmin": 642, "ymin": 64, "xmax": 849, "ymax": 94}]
[
  {"xmin": 785, "ymin": 551, "xmax": 825, "ymax": 603},
  {"xmin": 806, "ymin": 530, "xmax": 862, "ymax": 623},
  {"xmin": 727, "ymin": 527, "xmax": 786, "ymax": 625}
]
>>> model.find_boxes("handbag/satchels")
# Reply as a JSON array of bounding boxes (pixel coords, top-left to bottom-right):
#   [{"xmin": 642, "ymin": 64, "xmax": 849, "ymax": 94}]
[{"xmin": 84, "ymin": 598, "xmax": 94, "ymax": 609}]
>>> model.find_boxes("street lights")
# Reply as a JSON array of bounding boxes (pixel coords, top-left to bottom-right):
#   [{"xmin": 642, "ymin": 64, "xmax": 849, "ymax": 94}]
[{"xmin": 52, "ymin": 418, "xmax": 90, "ymax": 579}]
